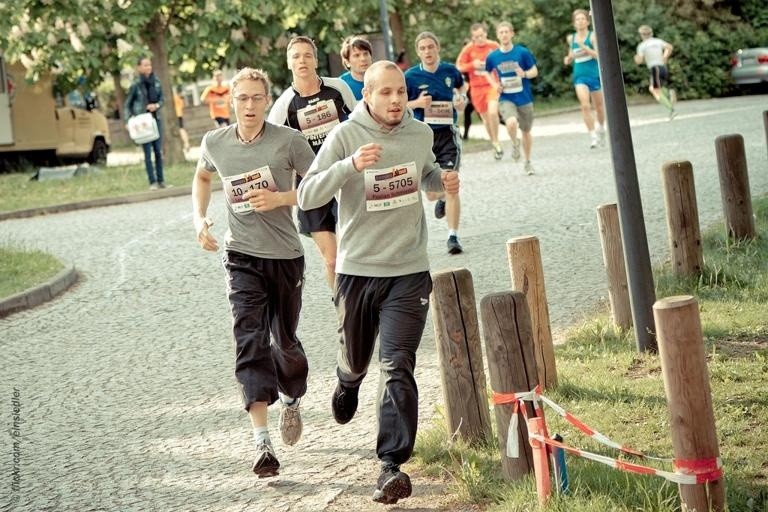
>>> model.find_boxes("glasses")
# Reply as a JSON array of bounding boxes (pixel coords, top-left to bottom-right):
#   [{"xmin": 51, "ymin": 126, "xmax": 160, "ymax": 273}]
[{"xmin": 232, "ymin": 93, "xmax": 268, "ymax": 104}]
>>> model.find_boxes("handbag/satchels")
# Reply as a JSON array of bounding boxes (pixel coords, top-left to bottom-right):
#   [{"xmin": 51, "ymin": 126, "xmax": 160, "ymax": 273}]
[{"xmin": 126, "ymin": 111, "xmax": 161, "ymax": 146}]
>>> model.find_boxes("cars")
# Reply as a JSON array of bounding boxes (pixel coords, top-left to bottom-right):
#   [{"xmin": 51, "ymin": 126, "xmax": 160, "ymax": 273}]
[{"xmin": 729, "ymin": 44, "xmax": 767, "ymax": 85}]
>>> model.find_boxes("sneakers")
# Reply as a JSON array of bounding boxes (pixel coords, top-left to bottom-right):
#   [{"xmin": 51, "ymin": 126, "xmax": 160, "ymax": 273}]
[
  {"xmin": 492, "ymin": 140, "xmax": 506, "ymax": 161},
  {"xmin": 148, "ymin": 182, "xmax": 175, "ymax": 191},
  {"xmin": 277, "ymin": 394, "xmax": 303, "ymax": 447},
  {"xmin": 524, "ymin": 162, "xmax": 534, "ymax": 178},
  {"xmin": 434, "ymin": 199, "xmax": 446, "ymax": 219},
  {"xmin": 331, "ymin": 379, "xmax": 360, "ymax": 425},
  {"xmin": 446, "ymin": 235, "xmax": 463, "ymax": 255},
  {"xmin": 510, "ymin": 138, "xmax": 521, "ymax": 161},
  {"xmin": 590, "ymin": 130, "xmax": 607, "ymax": 150},
  {"xmin": 371, "ymin": 462, "xmax": 412, "ymax": 505},
  {"xmin": 251, "ymin": 445, "xmax": 281, "ymax": 480}
]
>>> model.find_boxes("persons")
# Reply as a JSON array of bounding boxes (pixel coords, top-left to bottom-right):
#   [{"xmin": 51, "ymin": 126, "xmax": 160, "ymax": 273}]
[
  {"xmin": 401, "ymin": 31, "xmax": 468, "ymax": 257},
  {"xmin": 631, "ymin": 25, "xmax": 680, "ymax": 122},
  {"xmin": 484, "ymin": 21, "xmax": 539, "ymax": 177},
  {"xmin": 456, "ymin": 24, "xmax": 504, "ymax": 159},
  {"xmin": 336, "ymin": 34, "xmax": 375, "ymax": 107},
  {"xmin": 455, "ymin": 87, "xmax": 506, "ymax": 142},
  {"xmin": 267, "ymin": 36, "xmax": 357, "ymax": 300},
  {"xmin": 200, "ymin": 70, "xmax": 232, "ymax": 130},
  {"xmin": 187, "ymin": 67, "xmax": 315, "ymax": 478},
  {"xmin": 562, "ymin": 8, "xmax": 606, "ymax": 151},
  {"xmin": 170, "ymin": 75, "xmax": 190, "ymax": 154},
  {"xmin": 291, "ymin": 60, "xmax": 450, "ymax": 505},
  {"xmin": 124, "ymin": 54, "xmax": 172, "ymax": 195}
]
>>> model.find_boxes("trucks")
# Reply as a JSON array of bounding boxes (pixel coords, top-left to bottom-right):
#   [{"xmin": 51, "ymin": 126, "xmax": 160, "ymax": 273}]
[{"xmin": 0, "ymin": 49, "xmax": 113, "ymax": 168}]
